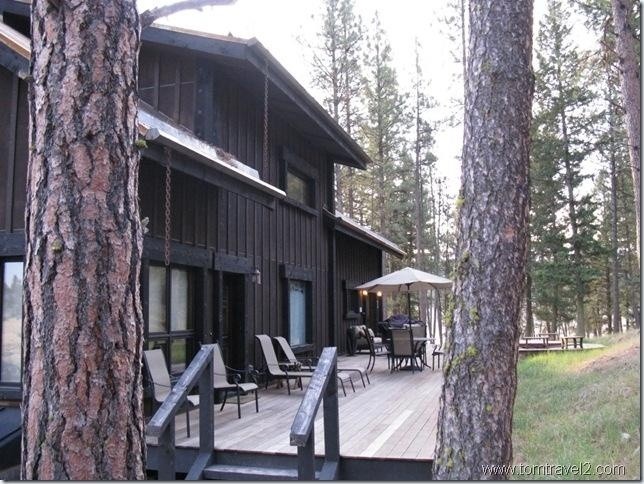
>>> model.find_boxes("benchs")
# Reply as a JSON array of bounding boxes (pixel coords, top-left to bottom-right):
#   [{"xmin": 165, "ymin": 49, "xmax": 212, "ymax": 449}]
[
  {"xmin": 561, "ymin": 335, "xmax": 584, "ymax": 349},
  {"xmin": 537, "ymin": 332, "xmax": 560, "ymax": 340},
  {"xmin": 522, "ymin": 336, "xmax": 549, "ymax": 348}
]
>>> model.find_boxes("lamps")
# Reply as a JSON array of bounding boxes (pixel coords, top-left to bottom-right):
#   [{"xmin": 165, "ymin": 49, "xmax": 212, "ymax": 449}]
[{"xmin": 251, "ymin": 265, "xmax": 262, "ymax": 285}]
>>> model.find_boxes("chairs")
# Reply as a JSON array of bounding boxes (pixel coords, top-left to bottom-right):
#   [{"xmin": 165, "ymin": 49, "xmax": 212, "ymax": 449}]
[
  {"xmin": 347, "ymin": 317, "xmax": 447, "ymax": 371},
  {"xmin": 143, "ymin": 347, "xmax": 198, "ymax": 437},
  {"xmin": 272, "ymin": 333, "xmax": 370, "ymax": 388},
  {"xmin": 199, "ymin": 341, "xmax": 267, "ymax": 420},
  {"xmin": 252, "ymin": 330, "xmax": 355, "ymax": 397}
]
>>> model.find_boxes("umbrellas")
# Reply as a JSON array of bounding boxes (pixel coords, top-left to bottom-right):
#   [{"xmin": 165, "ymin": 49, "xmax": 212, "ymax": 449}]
[{"xmin": 353, "ymin": 266, "xmax": 453, "ymax": 371}]
